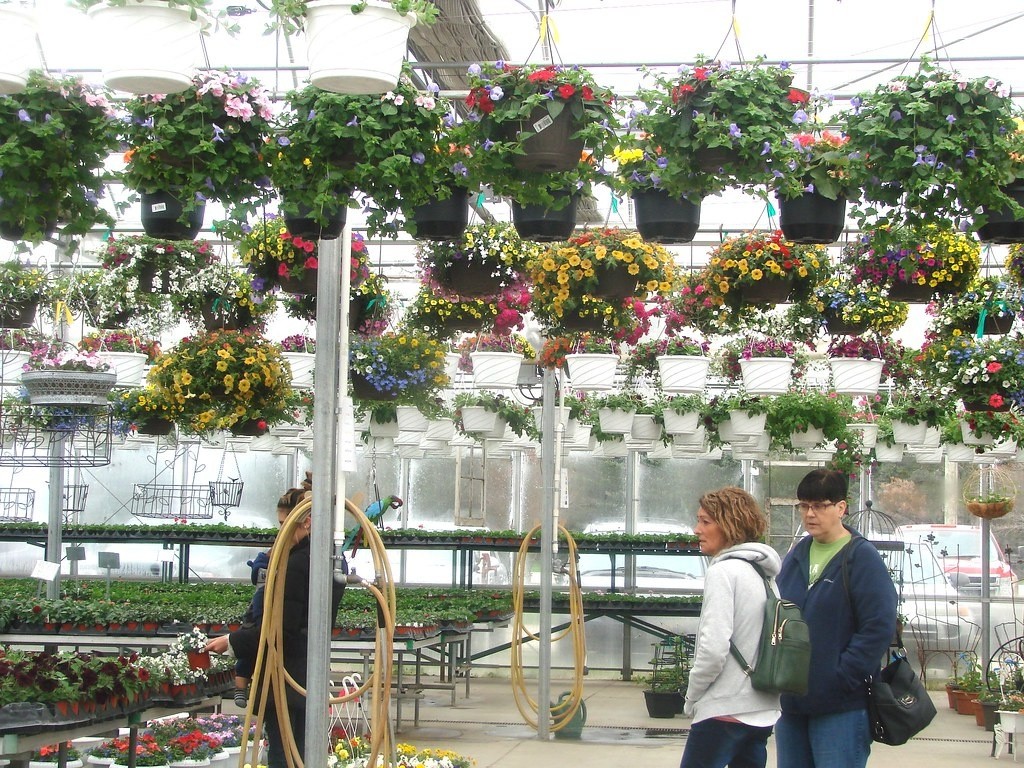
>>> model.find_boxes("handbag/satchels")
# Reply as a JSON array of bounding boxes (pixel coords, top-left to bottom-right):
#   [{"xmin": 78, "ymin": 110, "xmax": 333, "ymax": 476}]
[{"xmin": 868, "ymin": 658, "xmax": 938, "ymax": 746}]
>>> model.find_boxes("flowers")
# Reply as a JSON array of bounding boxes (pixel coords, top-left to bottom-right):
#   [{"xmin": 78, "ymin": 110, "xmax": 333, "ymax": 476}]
[
  {"xmin": 945, "ymin": 656, "xmax": 1024, "ymax": 710},
  {"xmin": 0, "ymin": 627, "xmax": 239, "ymax": 704},
  {"xmin": 31, "ymin": 713, "xmax": 263, "ymax": 768},
  {"xmin": 1, "ymin": 57, "xmax": 1024, "ymax": 481},
  {"xmin": 328, "ymin": 728, "xmax": 474, "ymax": 768}
]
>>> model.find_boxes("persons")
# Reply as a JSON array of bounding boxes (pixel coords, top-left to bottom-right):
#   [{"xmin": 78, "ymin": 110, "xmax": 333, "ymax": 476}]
[
  {"xmin": 680, "ymin": 488, "xmax": 782, "ymax": 768},
  {"xmin": 775, "ymin": 467, "xmax": 898, "ymax": 768},
  {"xmin": 234, "ymin": 546, "xmax": 296, "ymax": 709},
  {"xmin": 204, "ymin": 472, "xmax": 348, "ymax": 768}
]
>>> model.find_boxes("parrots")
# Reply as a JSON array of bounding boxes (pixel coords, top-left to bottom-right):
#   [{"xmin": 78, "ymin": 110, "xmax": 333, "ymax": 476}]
[{"xmin": 341, "ymin": 494, "xmax": 403, "ymax": 558}]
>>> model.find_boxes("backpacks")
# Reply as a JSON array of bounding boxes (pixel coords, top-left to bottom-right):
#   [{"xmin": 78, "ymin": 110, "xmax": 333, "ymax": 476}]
[{"xmin": 704, "ymin": 557, "xmax": 811, "ymax": 696}]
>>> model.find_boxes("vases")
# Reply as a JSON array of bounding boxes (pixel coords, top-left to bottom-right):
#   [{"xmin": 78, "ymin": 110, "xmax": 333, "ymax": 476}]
[
  {"xmin": 946, "ymin": 685, "xmax": 1024, "ymax": 762},
  {"xmin": 110, "ymin": 760, "xmax": 170, "ymax": 768},
  {"xmin": 244, "ymin": 738, "xmax": 264, "ymax": 766},
  {"xmin": 87, "ymin": 755, "xmax": 117, "ymax": 768},
  {"xmin": 31, "ymin": 758, "xmax": 81, "ymax": 768},
  {"xmin": 1, "ymin": 0, "xmax": 1024, "ymax": 466},
  {"xmin": 210, "ymin": 751, "xmax": 229, "ymax": 766},
  {"xmin": 172, "ymin": 755, "xmax": 210, "ymax": 767},
  {"xmin": 57, "ymin": 652, "xmax": 237, "ymax": 716},
  {"xmin": 224, "ymin": 748, "xmax": 242, "ymax": 766}
]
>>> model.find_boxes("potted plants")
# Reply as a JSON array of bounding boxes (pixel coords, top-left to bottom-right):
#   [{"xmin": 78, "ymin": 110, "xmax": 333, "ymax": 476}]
[
  {"xmin": 0, "ymin": 578, "xmax": 706, "ymax": 636},
  {"xmin": 643, "ymin": 638, "xmax": 678, "ymax": 718},
  {"xmin": 675, "ymin": 638, "xmax": 688, "ymax": 715},
  {"xmin": 960, "ymin": 466, "xmax": 1017, "ymax": 519},
  {"xmin": 2, "ymin": 518, "xmax": 732, "ymax": 559}
]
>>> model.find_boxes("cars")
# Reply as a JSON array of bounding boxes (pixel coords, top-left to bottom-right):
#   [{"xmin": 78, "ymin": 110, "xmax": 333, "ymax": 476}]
[
  {"xmin": 344, "ymin": 521, "xmax": 509, "ymax": 585},
  {"xmin": 0, "ymin": 514, "xmax": 274, "ymax": 577},
  {"xmin": 876, "ymin": 543, "xmax": 975, "ymax": 671},
  {"xmin": 562, "ymin": 521, "xmax": 709, "ymax": 597},
  {"xmin": 893, "ymin": 524, "xmax": 1020, "ymax": 597}
]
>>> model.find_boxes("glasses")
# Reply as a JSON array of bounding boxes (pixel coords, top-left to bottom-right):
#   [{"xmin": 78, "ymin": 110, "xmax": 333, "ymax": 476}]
[{"xmin": 795, "ymin": 503, "xmax": 826, "ymax": 514}]
